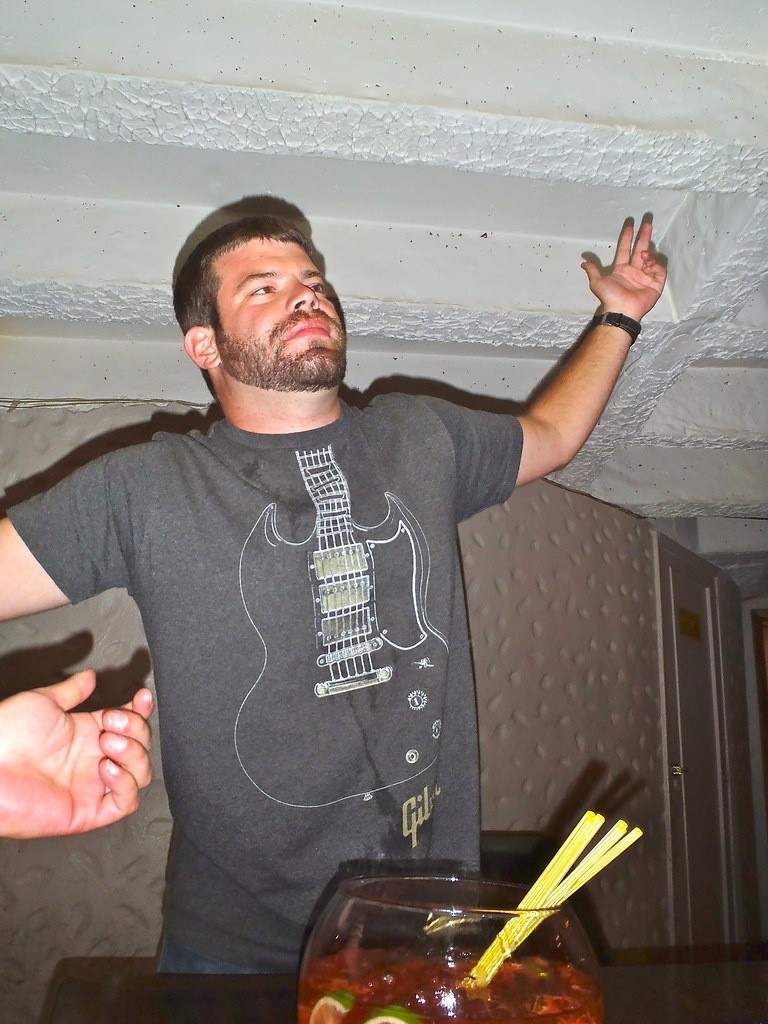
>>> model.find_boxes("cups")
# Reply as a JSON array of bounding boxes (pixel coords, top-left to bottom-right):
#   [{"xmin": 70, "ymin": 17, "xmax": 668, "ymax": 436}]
[{"xmin": 296, "ymin": 872, "xmax": 605, "ymax": 1024}]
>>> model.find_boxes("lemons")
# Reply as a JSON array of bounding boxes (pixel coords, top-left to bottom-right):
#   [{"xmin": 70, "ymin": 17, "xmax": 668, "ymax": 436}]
[
  {"xmin": 308, "ymin": 990, "xmax": 356, "ymax": 1024},
  {"xmin": 361, "ymin": 1004, "xmax": 423, "ymax": 1024}
]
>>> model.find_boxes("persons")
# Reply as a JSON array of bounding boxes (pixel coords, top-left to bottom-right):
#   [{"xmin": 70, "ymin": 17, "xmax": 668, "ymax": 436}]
[
  {"xmin": 0, "ymin": 215, "xmax": 669, "ymax": 978},
  {"xmin": 0, "ymin": 668, "xmax": 153, "ymax": 841}
]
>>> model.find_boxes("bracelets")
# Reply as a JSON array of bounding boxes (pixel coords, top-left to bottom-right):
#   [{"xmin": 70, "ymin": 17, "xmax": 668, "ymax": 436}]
[{"xmin": 592, "ymin": 311, "xmax": 641, "ymax": 346}]
